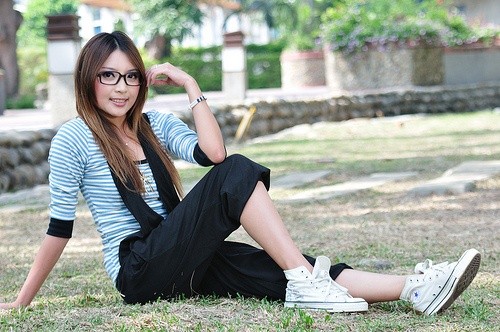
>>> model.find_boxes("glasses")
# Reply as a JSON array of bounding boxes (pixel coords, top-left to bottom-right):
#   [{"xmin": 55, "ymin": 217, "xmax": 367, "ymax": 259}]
[{"xmin": 95, "ymin": 71, "xmax": 143, "ymax": 86}]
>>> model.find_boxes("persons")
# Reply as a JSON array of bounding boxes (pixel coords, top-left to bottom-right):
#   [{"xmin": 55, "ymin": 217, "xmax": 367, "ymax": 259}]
[{"xmin": 0, "ymin": 29, "xmax": 481, "ymax": 320}]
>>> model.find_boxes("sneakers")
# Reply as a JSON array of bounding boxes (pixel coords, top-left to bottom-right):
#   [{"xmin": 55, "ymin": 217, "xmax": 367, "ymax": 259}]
[
  {"xmin": 282, "ymin": 254, "xmax": 368, "ymax": 312},
  {"xmin": 408, "ymin": 248, "xmax": 481, "ymax": 315}
]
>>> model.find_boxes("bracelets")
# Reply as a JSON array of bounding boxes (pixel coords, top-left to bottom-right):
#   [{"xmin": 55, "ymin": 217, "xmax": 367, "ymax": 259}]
[{"xmin": 187, "ymin": 95, "xmax": 208, "ymax": 110}]
